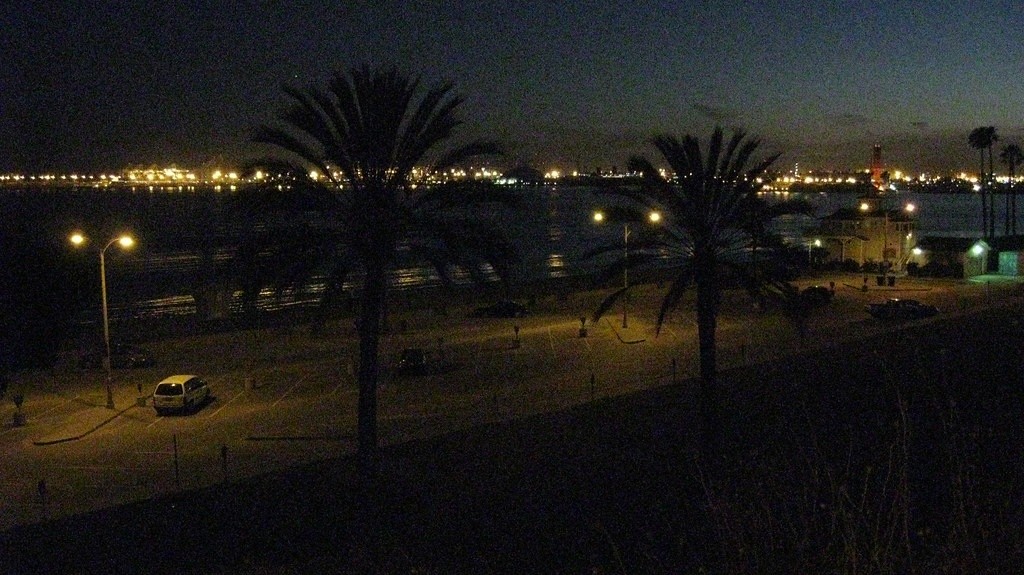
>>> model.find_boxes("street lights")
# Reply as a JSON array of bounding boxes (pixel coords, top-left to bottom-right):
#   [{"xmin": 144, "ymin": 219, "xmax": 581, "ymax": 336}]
[
  {"xmin": 593, "ymin": 208, "xmax": 662, "ymax": 326},
  {"xmin": 69, "ymin": 233, "xmax": 134, "ymax": 404},
  {"xmin": 859, "ymin": 202, "xmax": 916, "ymax": 265}
]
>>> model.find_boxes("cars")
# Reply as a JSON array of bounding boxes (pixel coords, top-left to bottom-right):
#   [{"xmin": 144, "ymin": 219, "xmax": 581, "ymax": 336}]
[
  {"xmin": 78, "ymin": 342, "xmax": 153, "ymax": 370},
  {"xmin": 398, "ymin": 348, "xmax": 428, "ymax": 375},
  {"xmin": 473, "ymin": 301, "xmax": 527, "ymax": 319},
  {"xmin": 799, "ymin": 286, "xmax": 831, "ymax": 307},
  {"xmin": 152, "ymin": 375, "xmax": 213, "ymax": 416},
  {"xmin": 864, "ymin": 298, "xmax": 940, "ymax": 324}
]
